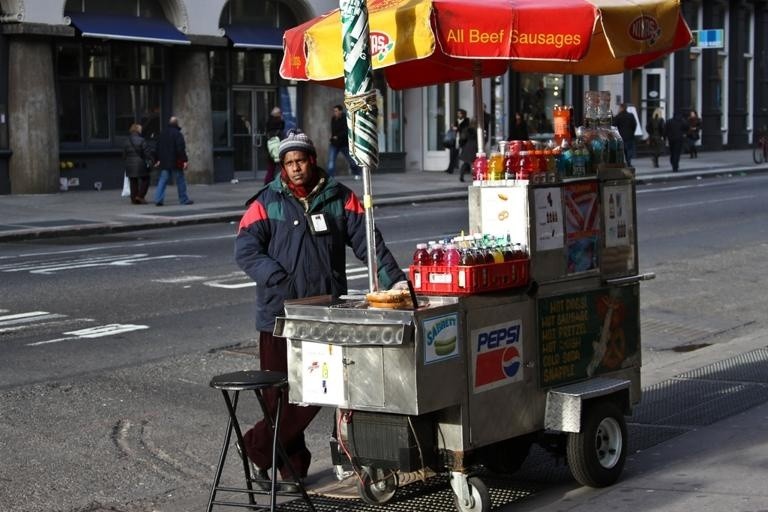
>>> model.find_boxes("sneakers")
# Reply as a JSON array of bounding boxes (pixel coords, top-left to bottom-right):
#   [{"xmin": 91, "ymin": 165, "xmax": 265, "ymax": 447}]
[
  {"xmin": 131, "ymin": 196, "xmax": 148, "ymax": 205},
  {"xmin": 155, "ymin": 202, "xmax": 165, "ymax": 206},
  {"xmin": 180, "ymin": 200, "xmax": 194, "ymax": 205},
  {"xmin": 353, "ymin": 174, "xmax": 362, "ymax": 181}
]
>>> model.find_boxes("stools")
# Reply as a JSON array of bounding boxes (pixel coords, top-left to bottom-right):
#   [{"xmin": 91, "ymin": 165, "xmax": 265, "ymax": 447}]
[{"xmin": 204, "ymin": 370, "xmax": 316, "ymax": 512}]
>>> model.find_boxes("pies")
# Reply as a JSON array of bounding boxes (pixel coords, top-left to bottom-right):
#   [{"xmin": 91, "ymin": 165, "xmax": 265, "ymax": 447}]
[{"xmin": 366, "ymin": 290, "xmax": 411, "ymax": 308}]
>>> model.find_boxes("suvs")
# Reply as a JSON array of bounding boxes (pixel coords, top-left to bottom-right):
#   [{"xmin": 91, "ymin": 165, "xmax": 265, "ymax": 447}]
[{"xmin": 213, "ymin": 111, "xmax": 248, "ymax": 147}]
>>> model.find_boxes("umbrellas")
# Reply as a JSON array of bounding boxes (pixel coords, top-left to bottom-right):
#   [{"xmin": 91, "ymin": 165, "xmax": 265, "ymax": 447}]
[
  {"xmin": 337, "ymin": 0, "xmax": 381, "ymax": 293},
  {"xmin": 277, "ymin": 0, "xmax": 696, "ymax": 155}
]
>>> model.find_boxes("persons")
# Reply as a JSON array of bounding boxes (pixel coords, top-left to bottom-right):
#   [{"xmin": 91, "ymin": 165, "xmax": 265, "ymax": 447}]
[
  {"xmin": 441, "ymin": 102, "xmax": 703, "ymax": 184},
  {"xmin": 522, "ymin": 78, "xmax": 533, "ymax": 133},
  {"xmin": 233, "ymin": 127, "xmax": 415, "ymax": 495},
  {"xmin": 262, "ymin": 106, "xmax": 288, "ymax": 188},
  {"xmin": 153, "ymin": 116, "xmax": 195, "ymax": 206},
  {"xmin": 324, "ymin": 104, "xmax": 362, "ymax": 182},
  {"xmin": 121, "ymin": 124, "xmax": 156, "ymax": 206},
  {"xmin": 534, "ymin": 80, "xmax": 549, "ymax": 133}
]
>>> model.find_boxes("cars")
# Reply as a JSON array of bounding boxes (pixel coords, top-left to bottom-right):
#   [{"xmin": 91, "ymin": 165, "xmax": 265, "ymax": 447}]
[{"xmin": 108, "ymin": 113, "xmax": 161, "ymax": 137}]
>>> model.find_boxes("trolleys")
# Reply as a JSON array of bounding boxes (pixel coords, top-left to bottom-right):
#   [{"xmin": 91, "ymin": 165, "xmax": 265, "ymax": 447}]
[{"xmin": 267, "ymin": 161, "xmax": 656, "ymax": 512}]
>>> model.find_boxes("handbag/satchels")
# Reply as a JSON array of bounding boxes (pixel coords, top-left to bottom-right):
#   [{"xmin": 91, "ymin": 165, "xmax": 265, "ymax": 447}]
[
  {"xmin": 173, "ymin": 157, "xmax": 188, "ymax": 170},
  {"xmin": 144, "ymin": 158, "xmax": 154, "ymax": 169}
]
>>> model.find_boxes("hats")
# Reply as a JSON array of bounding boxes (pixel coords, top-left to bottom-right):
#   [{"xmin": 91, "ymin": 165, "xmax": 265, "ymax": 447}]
[{"xmin": 275, "ymin": 127, "xmax": 318, "ymax": 161}]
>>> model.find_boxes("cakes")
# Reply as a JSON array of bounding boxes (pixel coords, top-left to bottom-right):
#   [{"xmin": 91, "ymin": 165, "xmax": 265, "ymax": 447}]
[{"xmin": 433, "ymin": 326, "xmax": 458, "ymax": 356}]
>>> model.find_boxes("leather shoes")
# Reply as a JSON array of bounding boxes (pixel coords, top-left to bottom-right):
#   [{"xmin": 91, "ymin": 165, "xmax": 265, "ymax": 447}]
[
  {"xmin": 233, "ymin": 437, "xmax": 273, "ymax": 492},
  {"xmin": 280, "ymin": 476, "xmax": 304, "ymax": 494}
]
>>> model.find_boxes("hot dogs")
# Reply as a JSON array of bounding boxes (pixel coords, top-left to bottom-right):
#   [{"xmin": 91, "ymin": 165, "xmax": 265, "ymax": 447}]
[{"xmin": 565, "ymin": 184, "xmax": 599, "ymax": 244}]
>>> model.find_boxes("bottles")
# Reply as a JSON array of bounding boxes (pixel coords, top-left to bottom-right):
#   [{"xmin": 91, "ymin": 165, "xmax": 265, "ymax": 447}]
[
  {"xmin": 583, "ymin": 89, "xmax": 613, "ymax": 130},
  {"xmin": 414, "ymin": 232, "xmax": 524, "ymax": 275},
  {"xmin": 471, "ymin": 126, "xmax": 627, "ymax": 183}
]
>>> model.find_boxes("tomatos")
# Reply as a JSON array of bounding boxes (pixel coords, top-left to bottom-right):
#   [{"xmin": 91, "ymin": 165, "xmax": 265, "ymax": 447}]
[{"xmin": 596, "ymin": 287, "xmax": 626, "ymax": 328}]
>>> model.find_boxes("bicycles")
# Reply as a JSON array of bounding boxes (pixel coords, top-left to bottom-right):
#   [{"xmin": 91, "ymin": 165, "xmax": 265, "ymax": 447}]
[{"xmin": 752, "ymin": 126, "xmax": 767, "ymax": 164}]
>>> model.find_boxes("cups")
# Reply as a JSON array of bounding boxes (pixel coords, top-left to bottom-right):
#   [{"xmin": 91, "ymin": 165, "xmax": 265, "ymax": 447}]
[{"xmin": 552, "ymin": 105, "xmax": 574, "ymax": 147}]
